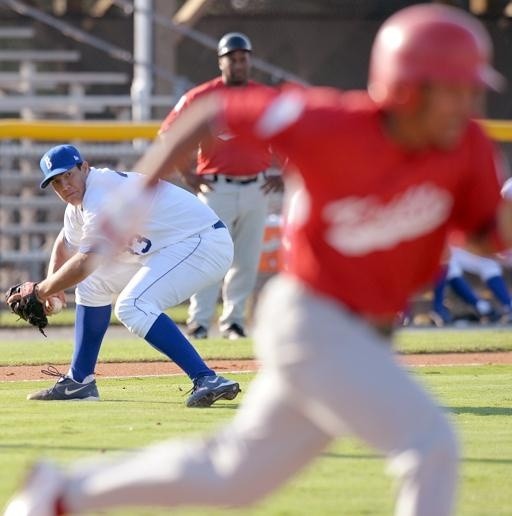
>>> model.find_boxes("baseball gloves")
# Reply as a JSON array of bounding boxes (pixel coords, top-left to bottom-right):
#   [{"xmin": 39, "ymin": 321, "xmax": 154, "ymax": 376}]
[{"xmin": 5, "ymin": 281, "xmax": 47, "ymax": 337}]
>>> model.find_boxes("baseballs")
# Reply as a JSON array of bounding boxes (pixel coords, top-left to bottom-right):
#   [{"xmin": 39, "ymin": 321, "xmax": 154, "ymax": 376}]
[{"xmin": 48, "ymin": 296, "xmax": 63, "ymax": 315}]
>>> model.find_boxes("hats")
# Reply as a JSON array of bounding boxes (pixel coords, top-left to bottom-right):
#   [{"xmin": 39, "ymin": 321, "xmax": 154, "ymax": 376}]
[{"xmin": 39, "ymin": 144, "xmax": 84, "ymax": 190}]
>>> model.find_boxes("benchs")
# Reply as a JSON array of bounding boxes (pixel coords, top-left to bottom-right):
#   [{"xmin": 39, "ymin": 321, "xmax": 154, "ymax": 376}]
[
  {"xmin": 1, "ymin": 142, "xmax": 151, "ymax": 281},
  {"xmin": 0, "ymin": 29, "xmax": 178, "ymax": 119}
]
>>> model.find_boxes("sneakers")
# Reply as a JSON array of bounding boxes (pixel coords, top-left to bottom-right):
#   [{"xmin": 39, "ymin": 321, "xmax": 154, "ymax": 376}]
[
  {"xmin": 222, "ymin": 324, "xmax": 245, "ymax": 341},
  {"xmin": 188, "ymin": 325, "xmax": 208, "ymax": 340},
  {"xmin": 0, "ymin": 460, "xmax": 69, "ymax": 516},
  {"xmin": 27, "ymin": 365, "xmax": 100, "ymax": 401},
  {"xmin": 181, "ymin": 372, "xmax": 242, "ymax": 409},
  {"xmin": 409, "ymin": 304, "xmax": 512, "ymax": 329}
]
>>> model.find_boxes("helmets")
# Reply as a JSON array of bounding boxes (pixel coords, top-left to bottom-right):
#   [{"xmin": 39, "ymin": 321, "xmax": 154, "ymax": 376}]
[
  {"xmin": 365, "ymin": 2, "xmax": 506, "ymax": 101},
  {"xmin": 215, "ymin": 32, "xmax": 254, "ymax": 58}
]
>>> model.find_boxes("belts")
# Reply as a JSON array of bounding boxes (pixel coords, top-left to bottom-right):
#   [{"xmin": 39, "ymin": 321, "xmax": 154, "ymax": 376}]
[{"xmin": 211, "ymin": 173, "xmax": 258, "ymax": 184}]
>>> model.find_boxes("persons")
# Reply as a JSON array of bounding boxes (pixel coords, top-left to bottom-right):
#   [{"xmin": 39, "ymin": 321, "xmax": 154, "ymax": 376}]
[
  {"xmin": 157, "ymin": 32, "xmax": 293, "ymax": 338},
  {"xmin": 6, "ymin": 144, "xmax": 239, "ymax": 407},
  {"xmin": 395, "ymin": 177, "xmax": 512, "ymax": 329},
  {"xmin": 0, "ymin": 3, "xmax": 512, "ymax": 516}
]
